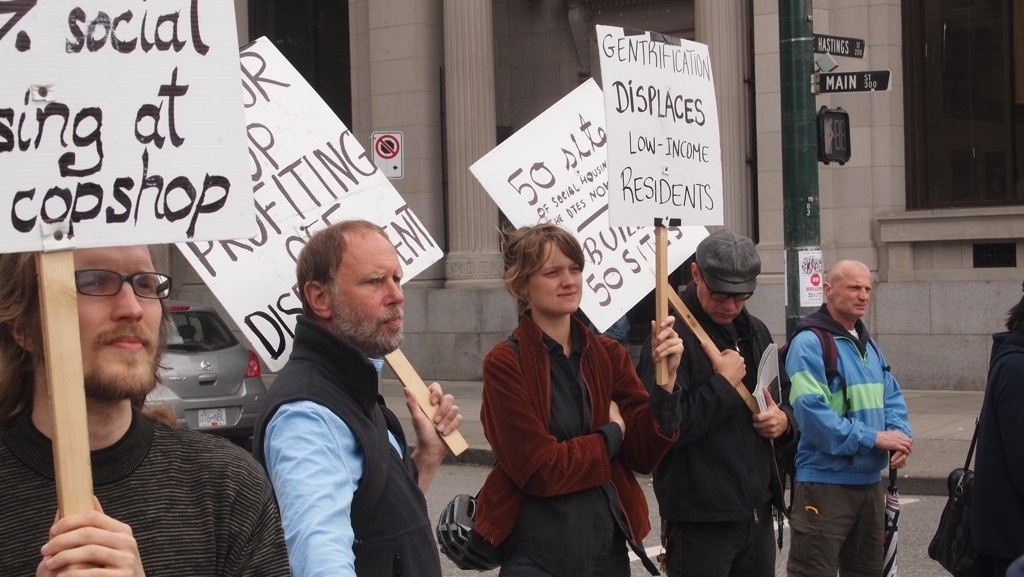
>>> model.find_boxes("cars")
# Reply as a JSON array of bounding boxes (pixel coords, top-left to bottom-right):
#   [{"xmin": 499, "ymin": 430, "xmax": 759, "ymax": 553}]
[{"xmin": 141, "ymin": 300, "xmax": 268, "ymax": 440}]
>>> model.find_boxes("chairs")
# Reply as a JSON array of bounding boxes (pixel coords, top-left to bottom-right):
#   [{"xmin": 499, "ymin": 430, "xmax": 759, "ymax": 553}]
[{"xmin": 177, "ymin": 325, "xmax": 196, "ymax": 343}]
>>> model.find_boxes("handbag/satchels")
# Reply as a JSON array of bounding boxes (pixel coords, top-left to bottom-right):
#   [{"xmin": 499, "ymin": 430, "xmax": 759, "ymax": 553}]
[
  {"xmin": 437, "ymin": 494, "xmax": 502, "ymax": 572},
  {"xmin": 928, "ymin": 468, "xmax": 1024, "ymax": 577}
]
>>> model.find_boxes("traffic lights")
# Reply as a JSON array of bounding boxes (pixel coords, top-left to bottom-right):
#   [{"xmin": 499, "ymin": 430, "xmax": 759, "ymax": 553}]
[{"xmin": 815, "ymin": 104, "xmax": 852, "ymax": 166}]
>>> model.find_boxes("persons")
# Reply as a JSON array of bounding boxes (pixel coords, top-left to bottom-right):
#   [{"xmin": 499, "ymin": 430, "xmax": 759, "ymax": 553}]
[
  {"xmin": 0, "ymin": 245, "xmax": 292, "ymax": 577},
  {"xmin": 587, "ymin": 312, "xmax": 630, "ymax": 353},
  {"xmin": 636, "ymin": 228, "xmax": 797, "ymax": 577},
  {"xmin": 473, "ymin": 224, "xmax": 684, "ymax": 577},
  {"xmin": 255, "ymin": 219, "xmax": 463, "ymax": 577},
  {"xmin": 978, "ymin": 276, "xmax": 1024, "ymax": 577},
  {"xmin": 787, "ymin": 260, "xmax": 912, "ymax": 577}
]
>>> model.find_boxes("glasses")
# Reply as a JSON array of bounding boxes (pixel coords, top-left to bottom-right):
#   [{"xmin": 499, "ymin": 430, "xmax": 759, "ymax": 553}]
[
  {"xmin": 75, "ymin": 268, "xmax": 171, "ymax": 298},
  {"xmin": 704, "ymin": 283, "xmax": 753, "ymax": 302}
]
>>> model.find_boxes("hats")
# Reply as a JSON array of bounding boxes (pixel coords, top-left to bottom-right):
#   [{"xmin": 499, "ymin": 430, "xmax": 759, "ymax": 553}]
[{"xmin": 696, "ymin": 229, "xmax": 761, "ymax": 294}]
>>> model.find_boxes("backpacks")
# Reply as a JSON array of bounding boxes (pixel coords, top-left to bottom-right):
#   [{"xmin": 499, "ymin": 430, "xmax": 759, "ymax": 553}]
[{"xmin": 769, "ymin": 327, "xmax": 837, "ymax": 475}]
